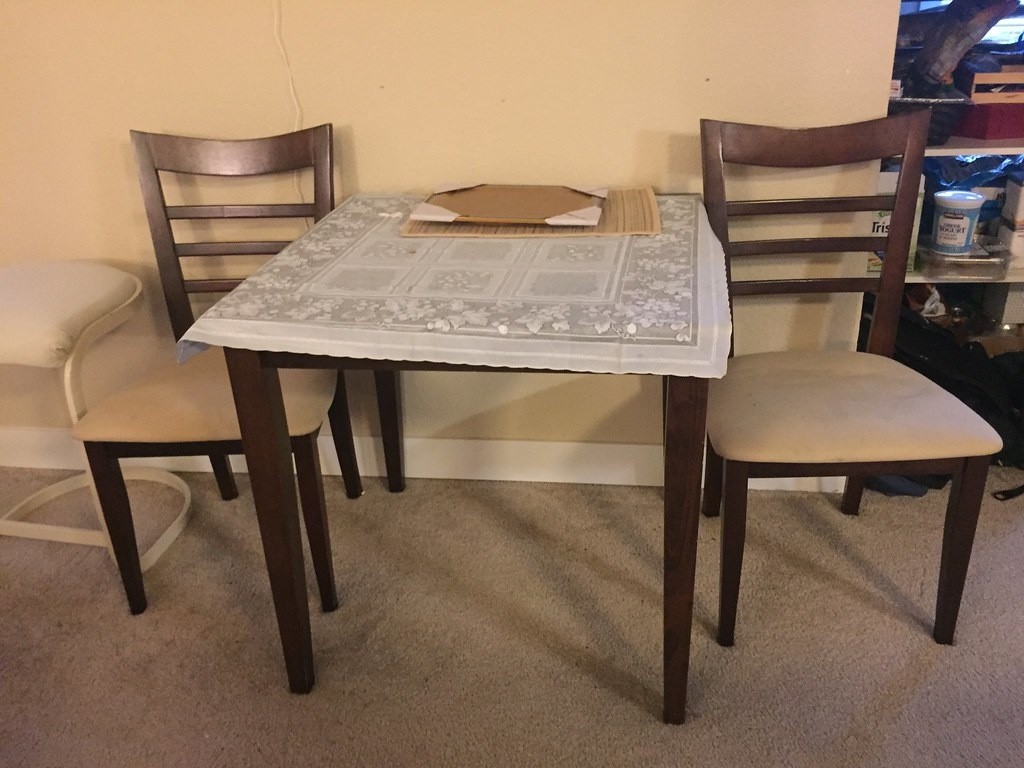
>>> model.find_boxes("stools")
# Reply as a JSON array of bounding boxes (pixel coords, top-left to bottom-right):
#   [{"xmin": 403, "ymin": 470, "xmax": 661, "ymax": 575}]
[{"xmin": 0, "ymin": 260, "xmax": 191, "ymax": 574}]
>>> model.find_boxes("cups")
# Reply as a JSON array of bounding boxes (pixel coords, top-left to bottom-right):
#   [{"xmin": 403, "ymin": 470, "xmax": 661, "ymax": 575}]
[{"xmin": 929, "ymin": 191, "xmax": 984, "ymax": 255}]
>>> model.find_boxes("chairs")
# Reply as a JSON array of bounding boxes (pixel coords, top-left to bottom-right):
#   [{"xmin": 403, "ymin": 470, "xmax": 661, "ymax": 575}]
[
  {"xmin": 702, "ymin": 110, "xmax": 1002, "ymax": 645},
  {"xmin": 69, "ymin": 121, "xmax": 362, "ymax": 619}
]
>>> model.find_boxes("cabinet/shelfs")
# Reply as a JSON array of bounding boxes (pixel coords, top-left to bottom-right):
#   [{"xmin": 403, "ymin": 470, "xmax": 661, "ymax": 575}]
[{"xmin": 857, "ymin": 0, "xmax": 1024, "ymax": 471}]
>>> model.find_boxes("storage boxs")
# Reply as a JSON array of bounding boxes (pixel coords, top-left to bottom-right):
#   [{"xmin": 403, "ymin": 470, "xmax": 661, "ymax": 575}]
[
  {"xmin": 997, "ymin": 178, "xmax": 1024, "ymax": 269},
  {"xmin": 865, "ymin": 171, "xmax": 925, "ymax": 272},
  {"xmin": 955, "ymin": 64, "xmax": 1023, "ymax": 104}
]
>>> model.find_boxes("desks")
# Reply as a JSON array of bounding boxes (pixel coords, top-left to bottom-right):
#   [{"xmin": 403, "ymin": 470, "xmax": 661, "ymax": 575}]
[{"xmin": 176, "ymin": 189, "xmax": 732, "ymax": 724}]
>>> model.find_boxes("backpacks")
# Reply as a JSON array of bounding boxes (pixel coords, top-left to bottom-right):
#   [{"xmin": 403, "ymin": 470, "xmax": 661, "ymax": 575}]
[{"xmin": 857, "ymin": 293, "xmax": 1023, "ymax": 499}]
